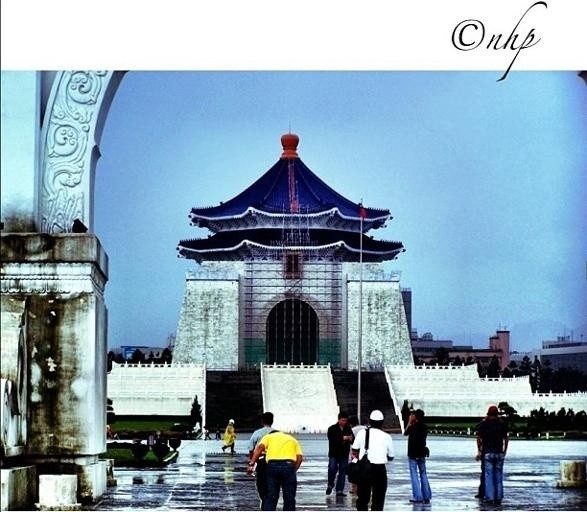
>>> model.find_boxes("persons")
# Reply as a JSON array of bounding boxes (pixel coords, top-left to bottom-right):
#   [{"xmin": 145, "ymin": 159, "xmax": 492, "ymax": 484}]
[
  {"xmin": 401, "ymin": 399, "xmax": 431, "ymax": 504},
  {"xmin": 221, "ymin": 419, "xmax": 236, "ymax": 452},
  {"xmin": 204, "ymin": 424, "xmax": 221, "ymax": 440},
  {"xmin": 475, "ymin": 406, "xmax": 508, "ymax": 505},
  {"xmin": 326, "ymin": 410, "xmax": 395, "ymax": 511},
  {"xmin": 247, "ymin": 413, "xmax": 303, "ymax": 511}
]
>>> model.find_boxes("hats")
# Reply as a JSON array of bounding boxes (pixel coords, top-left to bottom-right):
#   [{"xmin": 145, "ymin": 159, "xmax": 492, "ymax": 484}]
[
  {"xmin": 229, "ymin": 419, "xmax": 234, "ymax": 424},
  {"xmin": 488, "ymin": 406, "xmax": 500, "ymax": 414},
  {"xmin": 370, "ymin": 410, "xmax": 385, "ymax": 421}
]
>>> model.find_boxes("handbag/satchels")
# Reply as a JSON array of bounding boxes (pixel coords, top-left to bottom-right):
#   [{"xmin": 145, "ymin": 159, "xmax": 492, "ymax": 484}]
[{"xmin": 347, "ymin": 453, "xmax": 370, "ymax": 484}]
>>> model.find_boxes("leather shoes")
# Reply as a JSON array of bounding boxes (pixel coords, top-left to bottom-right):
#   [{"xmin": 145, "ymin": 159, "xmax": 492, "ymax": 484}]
[
  {"xmin": 336, "ymin": 492, "xmax": 347, "ymax": 497},
  {"xmin": 474, "ymin": 494, "xmax": 501, "ymax": 505},
  {"xmin": 326, "ymin": 483, "xmax": 335, "ymax": 495},
  {"xmin": 409, "ymin": 499, "xmax": 430, "ymax": 504}
]
